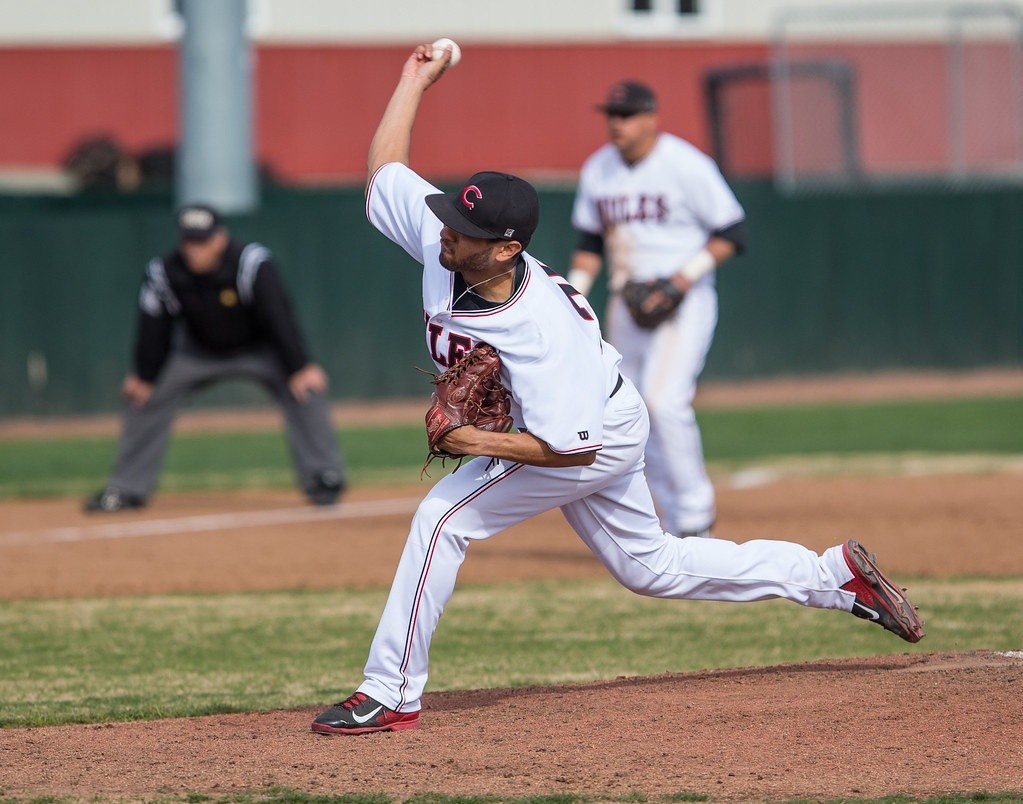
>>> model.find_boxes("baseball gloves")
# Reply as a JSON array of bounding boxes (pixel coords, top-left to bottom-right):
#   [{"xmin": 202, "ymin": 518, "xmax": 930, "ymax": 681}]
[
  {"xmin": 424, "ymin": 341, "xmax": 513, "ymax": 460},
  {"xmin": 622, "ymin": 278, "xmax": 686, "ymax": 334}
]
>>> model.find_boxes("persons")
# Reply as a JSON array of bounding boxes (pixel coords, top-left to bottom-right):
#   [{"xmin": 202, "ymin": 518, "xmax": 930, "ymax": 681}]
[
  {"xmin": 312, "ymin": 44, "xmax": 923, "ymax": 736},
  {"xmin": 566, "ymin": 78, "xmax": 746, "ymax": 539},
  {"xmin": 86, "ymin": 203, "xmax": 344, "ymax": 510}
]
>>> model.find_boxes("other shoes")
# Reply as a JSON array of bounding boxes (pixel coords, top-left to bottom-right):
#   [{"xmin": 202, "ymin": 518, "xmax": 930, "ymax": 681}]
[
  {"xmin": 84, "ymin": 489, "xmax": 139, "ymax": 509},
  {"xmin": 306, "ymin": 468, "xmax": 341, "ymax": 503}
]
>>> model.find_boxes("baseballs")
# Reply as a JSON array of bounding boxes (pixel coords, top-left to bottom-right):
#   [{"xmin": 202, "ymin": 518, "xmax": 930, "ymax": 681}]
[{"xmin": 430, "ymin": 37, "xmax": 462, "ymax": 69}]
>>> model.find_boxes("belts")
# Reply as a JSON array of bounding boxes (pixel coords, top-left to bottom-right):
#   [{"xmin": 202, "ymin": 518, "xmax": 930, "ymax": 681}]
[{"xmin": 609, "ymin": 373, "xmax": 624, "ymax": 399}]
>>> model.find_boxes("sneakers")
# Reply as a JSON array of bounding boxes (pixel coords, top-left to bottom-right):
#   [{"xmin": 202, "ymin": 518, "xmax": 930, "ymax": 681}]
[
  {"xmin": 839, "ymin": 539, "xmax": 924, "ymax": 644},
  {"xmin": 312, "ymin": 692, "xmax": 419, "ymax": 736}
]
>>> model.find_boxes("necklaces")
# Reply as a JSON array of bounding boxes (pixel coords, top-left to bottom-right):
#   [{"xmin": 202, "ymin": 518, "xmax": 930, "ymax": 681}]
[{"xmin": 452, "ymin": 265, "xmax": 517, "ymax": 307}]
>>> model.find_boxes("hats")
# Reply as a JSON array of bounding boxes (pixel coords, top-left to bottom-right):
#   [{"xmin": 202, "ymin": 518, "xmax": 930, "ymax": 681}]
[
  {"xmin": 595, "ymin": 81, "xmax": 655, "ymax": 112},
  {"xmin": 424, "ymin": 171, "xmax": 539, "ymax": 241},
  {"xmin": 176, "ymin": 206, "xmax": 217, "ymax": 241}
]
